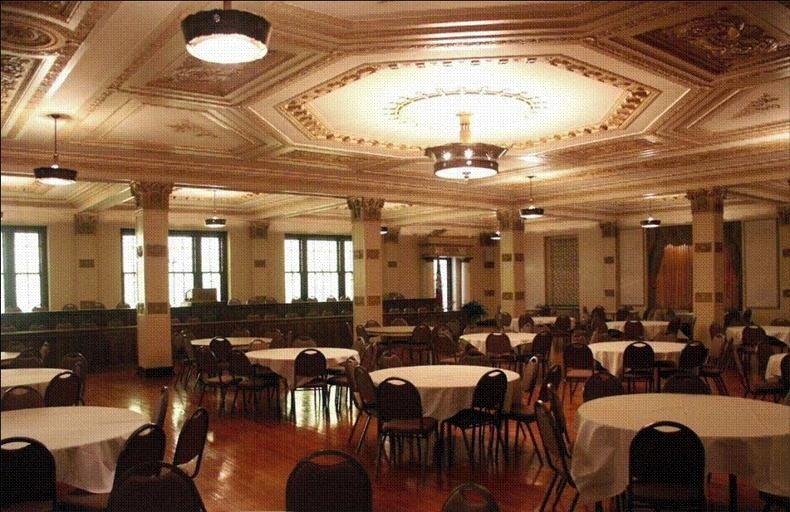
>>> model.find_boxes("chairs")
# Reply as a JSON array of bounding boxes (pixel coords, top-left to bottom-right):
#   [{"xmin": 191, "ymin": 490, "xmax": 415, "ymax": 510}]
[
  {"xmin": 609, "ymin": 419, "xmax": 706, "ymax": 511},
  {"xmin": 346, "ymin": 355, "xmax": 392, "ymax": 443},
  {"xmin": 162, "ymin": 406, "xmax": 210, "ymax": 477},
  {"xmin": 3, "ymin": 289, "xmax": 787, "ymax": 415},
  {"xmin": 535, "ymin": 400, "xmax": 605, "ymax": 509},
  {"xmin": 377, "ymin": 378, "xmax": 437, "ymax": 484},
  {"xmin": 487, "ymin": 364, "xmax": 560, "ymax": 465},
  {"xmin": 442, "ymin": 483, "xmax": 499, "ymax": 510},
  {"xmin": 153, "ymin": 386, "xmax": 172, "ymax": 431},
  {"xmin": 111, "ymin": 423, "xmax": 166, "ymax": 491},
  {"xmin": 353, "ymin": 364, "xmax": 404, "ymax": 453},
  {"xmin": 2, "ymin": 436, "xmax": 57, "ymax": 508},
  {"xmin": 491, "ymin": 356, "xmax": 540, "ymax": 455},
  {"xmin": 109, "ymin": 460, "xmax": 206, "ymax": 510},
  {"xmin": 440, "ymin": 369, "xmax": 507, "ymax": 470},
  {"xmin": 286, "ymin": 448, "xmax": 375, "ymax": 508}
]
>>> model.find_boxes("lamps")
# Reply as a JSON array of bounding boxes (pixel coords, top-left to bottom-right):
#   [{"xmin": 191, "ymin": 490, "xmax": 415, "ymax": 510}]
[
  {"xmin": 491, "ymin": 221, "xmax": 501, "ymax": 240},
  {"xmin": 640, "ymin": 189, "xmax": 661, "ymax": 229},
  {"xmin": 205, "ymin": 189, "xmax": 227, "ymax": 228},
  {"xmin": 519, "ymin": 175, "xmax": 544, "ymax": 219},
  {"xmin": 180, "ymin": 2, "xmax": 274, "ymax": 66},
  {"xmin": 422, "ymin": 110, "xmax": 509, "ymax": 196},
  {"xmin": 33, "ymin": 110, "xmax": 79, "ymax": 188}
]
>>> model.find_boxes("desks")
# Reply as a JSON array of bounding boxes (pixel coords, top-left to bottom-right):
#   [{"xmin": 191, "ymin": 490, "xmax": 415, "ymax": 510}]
[
  {"xmin": 571, "ymin": 392, "xmax": 789, "ymax": 510},
  {"xmin": 1, "ymin": 404, "xmax": 152, "ymax": 511}
]
